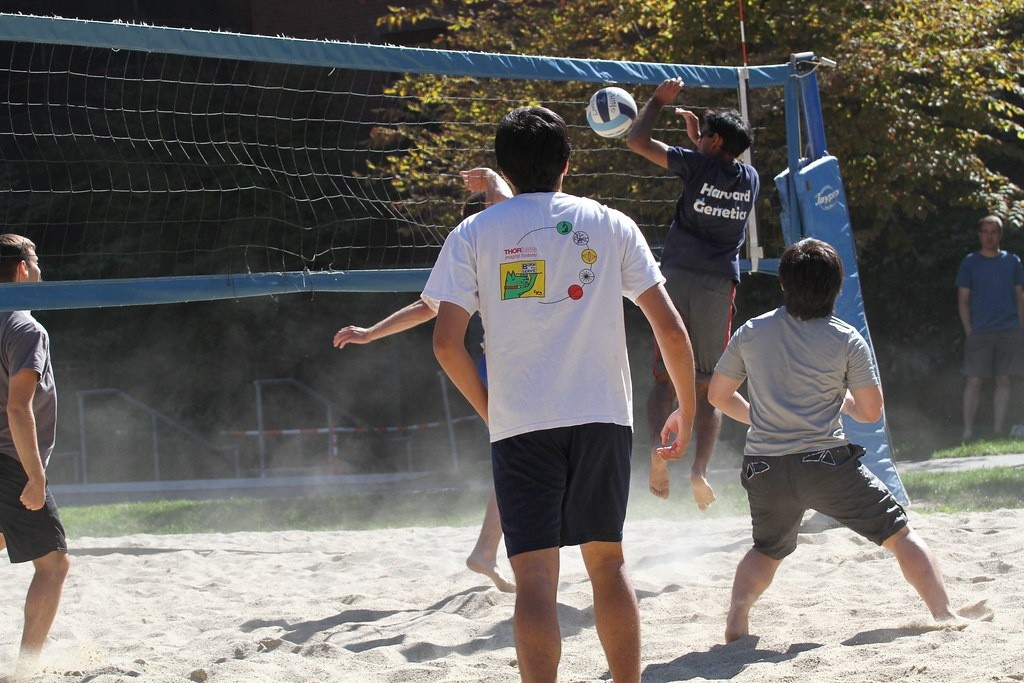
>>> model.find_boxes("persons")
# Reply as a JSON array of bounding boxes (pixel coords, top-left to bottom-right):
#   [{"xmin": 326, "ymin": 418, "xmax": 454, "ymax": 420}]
[
  {"xmin": 707, "ymin": 236, "xmax": 994, "ymax": 644},
  {"xmin": 954, "ymin": 215, "xmax": 1024, "ymax": 442},
  {"xmin": 333, "ymin": 167, "xmax": 516, "ymax": 594},
  {"xmin": 626, "ymin": 76, "xmax": 760, "ymax": 511},
  {"xmin": 420, "ymin": 104, "xmax": 697, "ymax": 682},
  {"xmin": 0, "ymin": 231, "xmax": 72, "ymax": 676}
]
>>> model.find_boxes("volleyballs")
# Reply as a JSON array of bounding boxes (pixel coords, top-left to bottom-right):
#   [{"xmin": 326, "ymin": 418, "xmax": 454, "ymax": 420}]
[{"xmin": 584, "ymin": 86, "xmax": 639, "ymax": 140}]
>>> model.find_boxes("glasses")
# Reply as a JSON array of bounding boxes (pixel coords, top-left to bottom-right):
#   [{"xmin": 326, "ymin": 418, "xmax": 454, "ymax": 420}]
[{"xmin": 699, "ymin": 133, "xmax": 705, "ymax": 140}]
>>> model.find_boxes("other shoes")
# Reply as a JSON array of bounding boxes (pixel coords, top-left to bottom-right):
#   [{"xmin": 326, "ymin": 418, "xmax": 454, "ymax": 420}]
[{"xmin": 958, "ymin": 431, "xmax": 978, "ymax": 447}]
